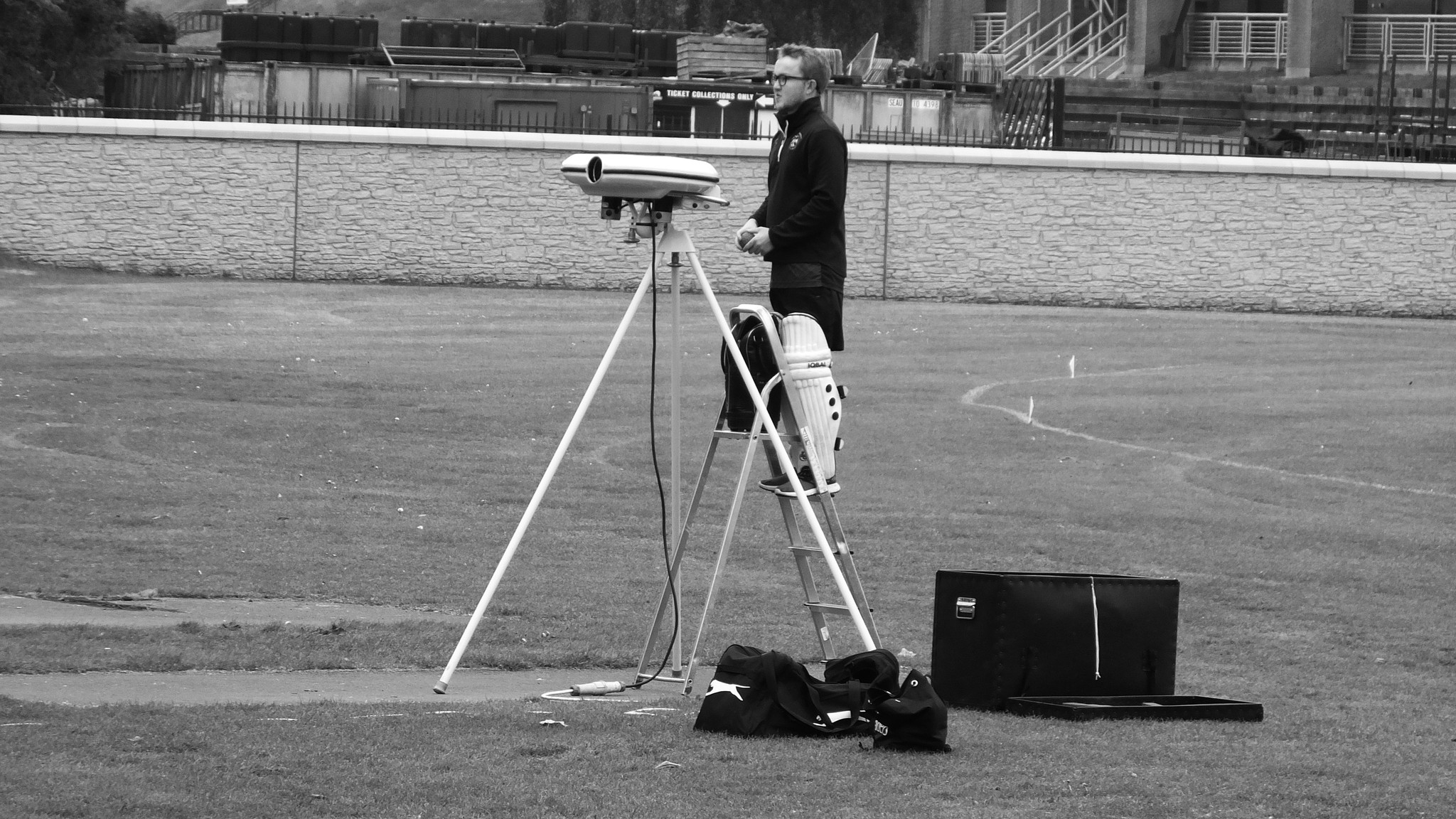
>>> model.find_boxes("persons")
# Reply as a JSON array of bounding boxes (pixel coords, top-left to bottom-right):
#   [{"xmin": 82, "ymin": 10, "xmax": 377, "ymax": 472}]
[{"xmin": 735, "ymin": 42, "xmax": 848, "ymax": 497}]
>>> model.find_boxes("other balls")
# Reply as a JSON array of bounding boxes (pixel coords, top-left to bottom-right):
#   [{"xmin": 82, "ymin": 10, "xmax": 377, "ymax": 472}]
[{"xmin": 739, "ymin": 230, "xmax": 755, "ymax": 251}]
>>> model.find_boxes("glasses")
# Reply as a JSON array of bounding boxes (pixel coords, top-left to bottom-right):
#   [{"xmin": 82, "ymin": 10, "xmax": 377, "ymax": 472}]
[{"xmin": 771, "ymin": 74, "xmax": 808, "ymax": 86}]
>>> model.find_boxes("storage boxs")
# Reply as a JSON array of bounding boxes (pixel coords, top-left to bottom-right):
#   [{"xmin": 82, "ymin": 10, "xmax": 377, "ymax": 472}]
[
  {"xmin": 400, "ymin": 16, "xmax": 766, "ymax": 82},
  {"xmin": 933, "ymin": 571, "xmax": 1263, "ymax": 720},
  {"xmin": 222, "ymin": 9, "xmax": 378, "ymax": 65}
]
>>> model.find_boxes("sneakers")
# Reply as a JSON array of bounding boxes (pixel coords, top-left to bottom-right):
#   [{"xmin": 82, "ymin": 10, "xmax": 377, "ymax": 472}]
[
  {"xmin": 758, "ymin": 466, "xmax": 811, "ymax": 491},
  {"xmin": 774, "ymin": 475, "xmax": 841, "ymax": 497}
]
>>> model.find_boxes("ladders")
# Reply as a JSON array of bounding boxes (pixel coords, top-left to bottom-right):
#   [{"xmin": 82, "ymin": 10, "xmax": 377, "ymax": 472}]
[{"xmin": 633, "ymin": 303, "xmax": 890, "ymax": 695}]
